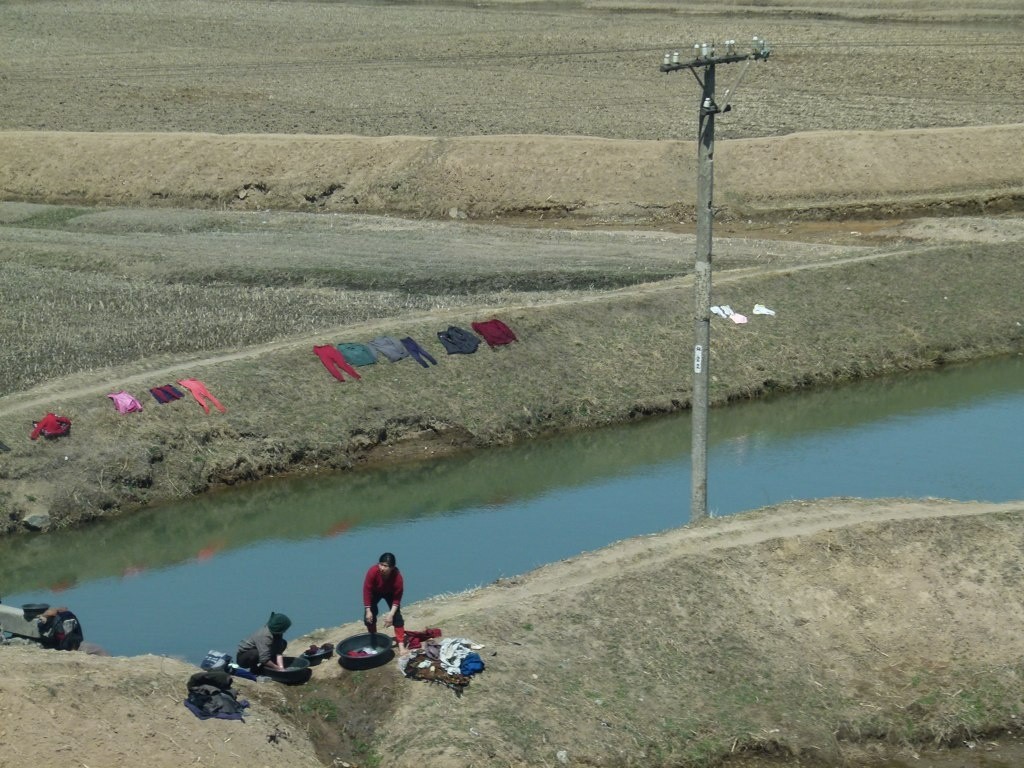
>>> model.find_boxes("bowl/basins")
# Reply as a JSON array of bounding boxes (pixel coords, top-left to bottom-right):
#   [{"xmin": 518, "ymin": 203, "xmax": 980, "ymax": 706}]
[
  {"xmin": 303, "ymin": 647, "xmax": 334, "ymax": 666},
  {"xmin": 262, "ymin": 657, "xmax": 310, "ymax": 684},
  {"xmin": 336, "ymin": 632, "xmax": 392, "ymax": 668},
  {"xmin": 22, "ymin": 604, "xmax": 50, "ymax": 616}
]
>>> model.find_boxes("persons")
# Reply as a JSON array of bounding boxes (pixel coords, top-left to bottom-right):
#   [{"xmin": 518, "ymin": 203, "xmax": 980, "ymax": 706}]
[
  {"xmin": 237, "ymin": 612, "xmax": 291, "ymax": 677},
  {"xmin": 363, "ymin": 551, "xmax": 406, "ymax": 655}
]
